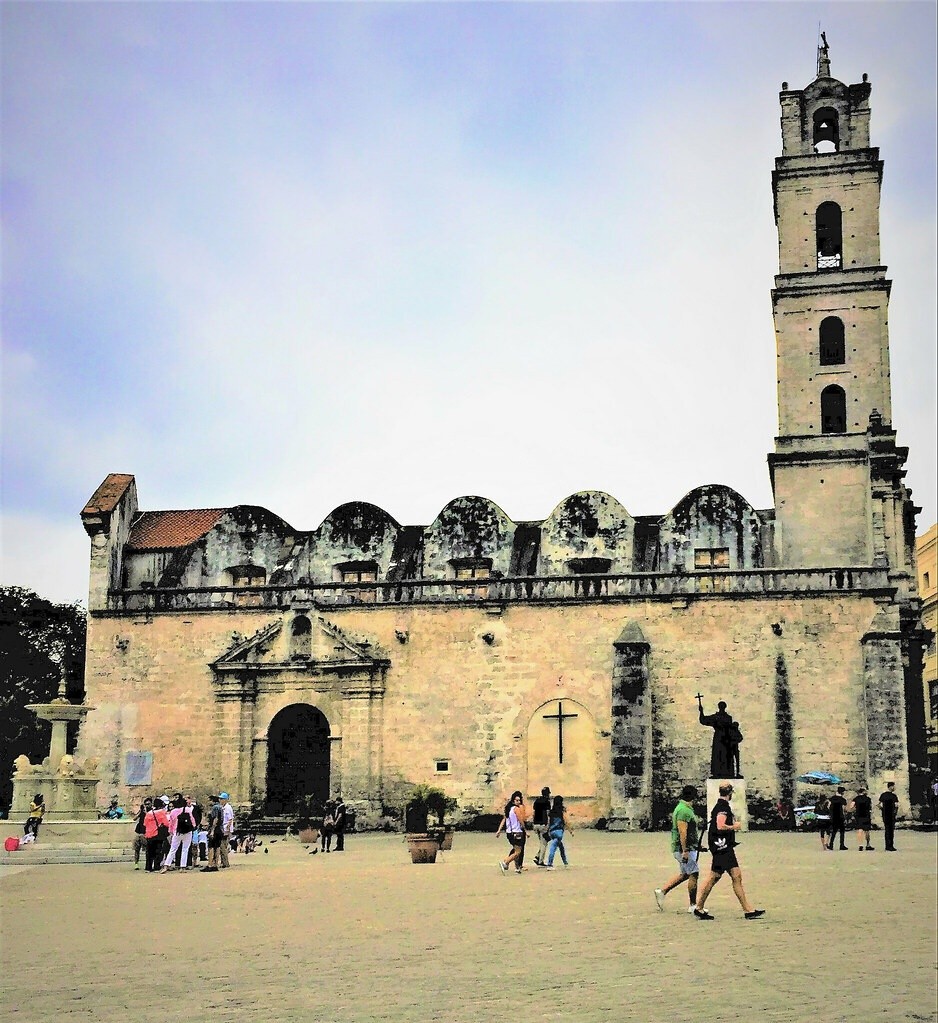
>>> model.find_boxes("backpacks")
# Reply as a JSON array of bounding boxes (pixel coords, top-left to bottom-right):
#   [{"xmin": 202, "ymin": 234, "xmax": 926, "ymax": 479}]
[{"xmin": 176, "ymin": 808, "xmax": 194, "ymax": 834}]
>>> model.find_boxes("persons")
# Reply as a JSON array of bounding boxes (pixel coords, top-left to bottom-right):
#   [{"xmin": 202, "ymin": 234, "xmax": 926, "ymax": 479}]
[
  {"xmin": 320, "ymin": 800, "xmax": 335, "ymax": 853},
  {"xmin": 654, "ymin": 781, "xmax": 765, "ymax": 919},
  {"xmin": 183, "ymin": 795, "xmax": 202, "ymax": 869},
  {"xmin": 850, "ymin": 788, "xmax": 875, "ymax": 851},
  {"xmin": 533, "ymin": 787, "xmax": 575, "ymax": 871},
  {"xmin": 172, "ymin": 793, "xmax": 182, "ymax": 801},
  {"xmin": 106, "ymin": 799, "xmax": 123, "ymax": 820},
  {"xmin": 143, "ymin": 798, "xmax": 169, "ymax": 873},
  {"xmin": 495, "ymin": 790, "xmax": 530, "ymax": 875},
  {"xmin": 876, "ymin": 781, "xmax": 899, "ymax": 851},
  {"xmin": 200, "ymin": 796, "xmax": 223, "ymax": 871},
  {"xmin": 24, "ymin": 794, "xmax": 45, "ymax": 844},
  {"xmin": 160, "ymin": 799, "xmax": 196, "ymax": 873},
  {"xmin": 931, "ymin": 778, "xmax": 938, "ymax": 806},
  {"xmin": 778, "ymin": 798, "xmax": 792, "ymax": 833},
  {"xmin": 213, "ymin": 793, "xmax": 234, "ymax": 868},
  {"xmin": 192, "ymin": 797, "xmax": 204, "ymax": 867},
  {"xmin": 133, "ymin": 798, "xmax": 153, "ymax": 870},
  {"xmin": 167, "ymin": 801, "xmax": 177, "ymax": 870},
  {"xmin": 699, "ymin": 701, "xmax": 744, "ymax": 776},
  {"xmin": 827, "ymin": 787, "xmax": 849, "ymax": 850},
  {"xmin": 333, "ymin": 797, "xmax": 347, "ymax": 851},
  {"xmin": 814, "ymin": 794, "xmax": 830, "ymax": 850}
]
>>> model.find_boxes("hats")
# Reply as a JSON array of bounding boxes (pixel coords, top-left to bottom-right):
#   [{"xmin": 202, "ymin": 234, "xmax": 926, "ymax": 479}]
[
  {"xmin": 218, "ymin": 793, "xmax": 228, "ymax": 799},
  {"xmin": 682, "ymin": 785, "xmax": 698, "ymax": 801},
  {"xmin": 719, "ymin": 783, "xmax": 734, "ymax": 795}
]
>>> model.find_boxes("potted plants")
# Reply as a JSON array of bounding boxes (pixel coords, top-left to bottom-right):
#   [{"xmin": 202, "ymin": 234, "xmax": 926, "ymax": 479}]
[
  {"xmin": 228, "ymin": 786, "xmax": 268, "ymax": 853},
  {"xmin": 405, "ymin": 784, "xmax": 460, "ymax": 863},
  {"xmin": 293, "ymin": 794, "xmax": 326, "ymax": 844}
]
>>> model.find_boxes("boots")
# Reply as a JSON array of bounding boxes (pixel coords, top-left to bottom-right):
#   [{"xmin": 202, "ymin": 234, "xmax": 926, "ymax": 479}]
[
  {"xmin": 821, "ymin": 838, "xmax": 826, "ymax": 850},
  {"xmin": 825, "ymin": 835, "xmax": 829, "ymax": 847}
]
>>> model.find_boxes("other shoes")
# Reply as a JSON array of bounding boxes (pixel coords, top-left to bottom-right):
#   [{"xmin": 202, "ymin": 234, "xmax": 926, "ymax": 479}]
[
  {"xmin": 886, "ymin": 846, "xmax": 896, "ymax": 851},
  {"xmin": 839, "ymin": 846, "xmax": 848, "ymax": 850},
  {"xmin": 865, "ymin": 845, "xmax": 874, "ymax": 850},
  {"xmin": 744, "ymin": 909, "xmax": 765, "ymax": 918},
  {"xmin": 828, "ymin": 842, "xmax": 833, "ymax": 849},
  {"xmin": 134, "ymin": 858, "xmax": 230, "ymax": 874},
  {"xmin": 694, "ymin": 909, "xmax": 715, "ymax": 920},
  {"xmin": 859, "ymin": 846, "xmax": 863, "ymax": 851}
]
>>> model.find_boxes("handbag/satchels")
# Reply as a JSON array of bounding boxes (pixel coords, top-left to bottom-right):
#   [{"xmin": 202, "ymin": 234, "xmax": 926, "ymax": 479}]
[
  {"xmin": 20, "ymin": 832, "xmax": 35, "ymax": 844},
  {"xmin": 513, "ymin": 833, "xmax": 524, "ymax": 845},
  {"xmin": 542, "ymin": 831, "xmax": 551, "ymax": 842},
  {"xmin": 5, "ymin": 836, "xmax": 18, "ymax": 851},
  {"xmin": 158, "ymin": 824, "xmax": 170, "ymax": 840}
]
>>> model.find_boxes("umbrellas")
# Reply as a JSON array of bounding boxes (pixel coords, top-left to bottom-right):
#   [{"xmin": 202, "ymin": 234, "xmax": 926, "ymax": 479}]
[{"xmin": 797, "ymin": 771, "xmax": 842, "ymax": 785}]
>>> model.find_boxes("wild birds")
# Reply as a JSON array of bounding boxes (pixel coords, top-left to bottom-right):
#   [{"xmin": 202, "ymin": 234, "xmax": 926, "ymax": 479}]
[
  {"xmin": 257, "ymin": 841, "xmax": 262, "ymax": 846},
  {"xmin": 264, "ymin": 848, "xmax": 268, "ymax": 854},
  {"xmin": 309, "ymin": 847, "xmax": 318, "ymax": 854},
  {"xmin": 303, "ymin": 845, "xmax": 309, "ymax": 849},
  {"xmin": 270, "ymin": 840, "xmax": 277, "ymax": 843}
]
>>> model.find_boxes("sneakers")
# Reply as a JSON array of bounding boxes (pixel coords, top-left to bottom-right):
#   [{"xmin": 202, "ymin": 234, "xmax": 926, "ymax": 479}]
[
  {"xmin": 499, "ymin": 856, "xmax": 571, "ymax": 875},
  {"xmin": 688, "ymin": 905, "xmax": 708, "ymax": 914},
  {"xmin": 655, "ymin": 889, "xmax": 665, "ymax": 910}
]
